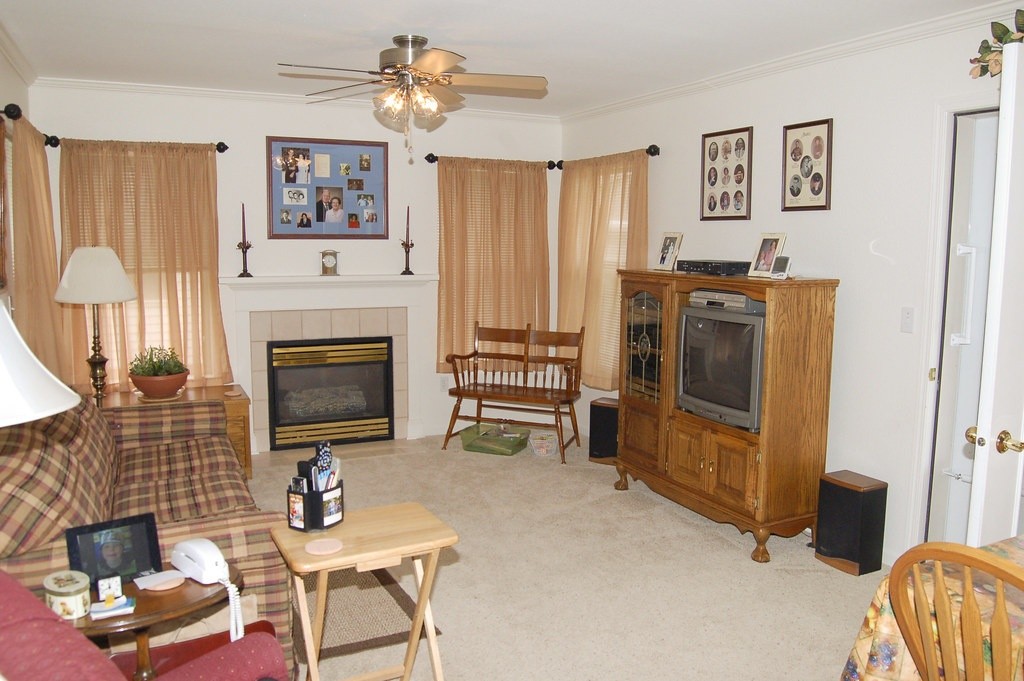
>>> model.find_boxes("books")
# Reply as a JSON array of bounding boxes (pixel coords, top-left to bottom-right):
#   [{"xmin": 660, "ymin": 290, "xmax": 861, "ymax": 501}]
[{"xmin": 90, "ymin": 596, "xmax": 136, "ymax": 621}]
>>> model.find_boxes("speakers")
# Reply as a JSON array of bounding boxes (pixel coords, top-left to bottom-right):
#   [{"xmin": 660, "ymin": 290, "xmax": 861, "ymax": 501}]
[
  {"xmin": 814, "ymin": 470, "xmax": 888, "ymax": 576},
  {"xmin": 588, "ymin": 397, "xmax": 619, "ymax": 466}
]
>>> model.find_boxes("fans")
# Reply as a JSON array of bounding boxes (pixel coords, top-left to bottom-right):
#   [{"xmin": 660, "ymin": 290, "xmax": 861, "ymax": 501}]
[{"xmin": 277, "ymin": 35, "xmax": 548, "ymax": 105}]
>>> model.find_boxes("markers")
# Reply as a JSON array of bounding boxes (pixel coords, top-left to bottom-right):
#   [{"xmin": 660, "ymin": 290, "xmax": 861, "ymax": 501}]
[{"xmin": 326, "ymin": 472, "xmax": 335, "ymax": 490}]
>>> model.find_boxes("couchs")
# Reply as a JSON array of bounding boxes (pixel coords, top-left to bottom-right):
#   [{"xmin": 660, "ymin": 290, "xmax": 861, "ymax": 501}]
[{"xmin": 0, "ymin": 385, "xmax": 294, "ymax": 680}]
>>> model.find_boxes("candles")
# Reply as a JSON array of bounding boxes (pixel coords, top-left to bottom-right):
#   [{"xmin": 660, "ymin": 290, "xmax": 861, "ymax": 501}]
[
  {"xmin": 242, "ymin": 203, "xmax": 246, "ymax": 245},
  {"xmin": 406, "ymin": 206, "xmax": 409, "ymax": 245}
]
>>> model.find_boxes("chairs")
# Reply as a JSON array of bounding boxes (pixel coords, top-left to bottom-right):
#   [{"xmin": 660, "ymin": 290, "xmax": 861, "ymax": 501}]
[{"xmin": 889, "ymin": 541, "xmax": 1024, "ymax": 681}]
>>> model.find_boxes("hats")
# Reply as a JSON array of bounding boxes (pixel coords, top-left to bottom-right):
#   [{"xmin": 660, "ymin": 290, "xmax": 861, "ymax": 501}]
[{"xmin": 99, "ymin": 529, "xmax": 125, "ymax": 550}]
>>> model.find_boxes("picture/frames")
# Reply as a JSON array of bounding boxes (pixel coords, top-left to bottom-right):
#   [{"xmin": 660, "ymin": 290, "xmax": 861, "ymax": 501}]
[
  {"xmin": 781, "ymin": 118, "xmax": 833, "ymax": 211},
  {"xmin": 699, "ymin": 126, "xmax": 753, "ymax": 221},
  {"xmin": 748, "ymin": 233, "xmax": 786, "ymax": 277},
  {"xmin": 654, "ymin": 232, "xmax": 684, "ymax": 270},
  {"xmin": 266, "ymin": 136, "xmax": 389, "ymax": 240},
  {"xmin": 65, "ymin": 513, "xmax": 162, "ymax": 591}
]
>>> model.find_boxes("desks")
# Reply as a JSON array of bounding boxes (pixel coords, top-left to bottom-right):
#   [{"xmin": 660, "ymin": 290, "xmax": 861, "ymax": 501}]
[
  {"xmin": 41, "ymin": 563, "xmax": 244, "ymax": 681},
  {"xmin": 270, "ymin": 502, "xmax": 458, "ymax": 681},
  {"xmin": 841, "ymin": 535, "xmax": 1024, "ymax": 681}
]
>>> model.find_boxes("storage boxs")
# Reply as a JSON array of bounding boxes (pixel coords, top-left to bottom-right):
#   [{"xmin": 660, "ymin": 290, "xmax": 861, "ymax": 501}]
[{"xmin": 459, "ymin": 424, "xmax": 531, "ymax": 455}]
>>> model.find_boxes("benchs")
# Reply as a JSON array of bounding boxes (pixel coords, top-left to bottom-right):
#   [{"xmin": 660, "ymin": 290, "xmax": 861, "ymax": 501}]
[{"xmin": 441, "ymin": 321, "xmax": 585, "ymax": 465}]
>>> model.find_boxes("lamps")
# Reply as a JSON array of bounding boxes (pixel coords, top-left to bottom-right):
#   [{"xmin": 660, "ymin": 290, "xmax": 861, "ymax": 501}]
[
  {"xmin": 0, "ymin": 298, "xmax": 81, "ymax": 426},
  {"xmin": 54, "ymin": 244, "xmax": 136, "ymax": 399},
  {"xmin": 373, "ymin": 70, "xmax": 444, "ymax": 153}
]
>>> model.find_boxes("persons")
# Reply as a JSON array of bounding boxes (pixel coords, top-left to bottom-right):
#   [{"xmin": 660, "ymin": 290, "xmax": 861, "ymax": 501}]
[
  {"xmin": 348, "ymin": 180, "xmax": 363, "ymax": 190},
  {"xmin": 364, "ymin": 211, "xmax": 377, "ymax": 222},
  {"xmin": 297, "ymin": 213, "xmax": 312, "ymax": 228},
  {"xmin": 348, "ymin": 214, "xmax": 360, "ymax": 228},
  {"xmin": 708, "ymin": 190, "xmax": 744, "ymax": 210},
  {"xmin": 282, "ymin": 148, "xmax": 309, "ymax": 183},
  {"xmin": 285, "ymin": 191, "xmax": 305, "ymax": 204},
  {"xmin": 791, "ymin": 136, "xmax": 824, "ymax": 161},
  {"xmin": 801, "ymin": 156, "xmax": 811, "ymax": 178},
  {"xmin": 281, "ymin": 210, "xmax": 291, "ymax": 224},
  {"xmin": 790, "ymin": 173, "xmax": 823, "ymax": 197},
  {"xmin": 709, "ymin": 138, "xmax": 745, "ymax": 161},
  {"xmin": 754, "ymin": 239, "xmax": 777, "ymax": 271},
  {"xmin": 708, "ymin": 164, "xmax": 744, "ymax": 186},
  {"xmin": 660, "ymin": 238, "xmax": 674, "ymax": 265},
  {"xmin": 316, "ymin": 189, "xmax": 343, "ymax": 223},
  {"xmin": 357, "ymin": 195, "xmax": 374, "ymax": 206},
  {"xmin": 97, "ymin": 528, "xmax": 136, "ymax": 577}
]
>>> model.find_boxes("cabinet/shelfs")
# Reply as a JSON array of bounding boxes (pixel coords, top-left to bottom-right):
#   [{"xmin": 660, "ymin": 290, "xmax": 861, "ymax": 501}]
[
  {"xmin": 613, "ymin": 269, "xmax": 840, "ymax": 562},
  {"xmin": 77, "ymin": 385, "xmax": 252, "ymax": 479}
]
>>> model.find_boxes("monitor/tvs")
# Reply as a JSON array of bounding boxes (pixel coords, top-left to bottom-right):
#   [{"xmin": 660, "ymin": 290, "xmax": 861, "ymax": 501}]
[{"xmin": 676, "ymin": 306, "xmax": 766, "ymax": 433}]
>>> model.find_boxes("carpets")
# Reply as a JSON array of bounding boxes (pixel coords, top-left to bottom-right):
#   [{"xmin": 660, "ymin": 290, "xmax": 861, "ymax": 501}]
[{"xmin": 291, "ymin": 567, "xmax": 442, "ymax": 666}]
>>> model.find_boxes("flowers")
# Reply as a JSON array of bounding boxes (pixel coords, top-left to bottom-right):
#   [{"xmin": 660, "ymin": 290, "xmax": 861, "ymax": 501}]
[{"xmin": 969, "ymin": 9, "xmax": 1024, "ymax": 79}]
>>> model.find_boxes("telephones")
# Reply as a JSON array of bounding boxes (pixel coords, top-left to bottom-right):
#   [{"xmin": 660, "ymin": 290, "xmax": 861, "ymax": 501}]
[{"xmin": 170, "ymin": 538, "xmax": 230, "ymax": 584}]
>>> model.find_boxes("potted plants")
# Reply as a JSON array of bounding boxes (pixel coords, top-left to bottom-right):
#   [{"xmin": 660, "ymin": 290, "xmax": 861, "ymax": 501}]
[{"xmin": 128, "ymin": 345, "xmax": 190, "ymax": 398}]
